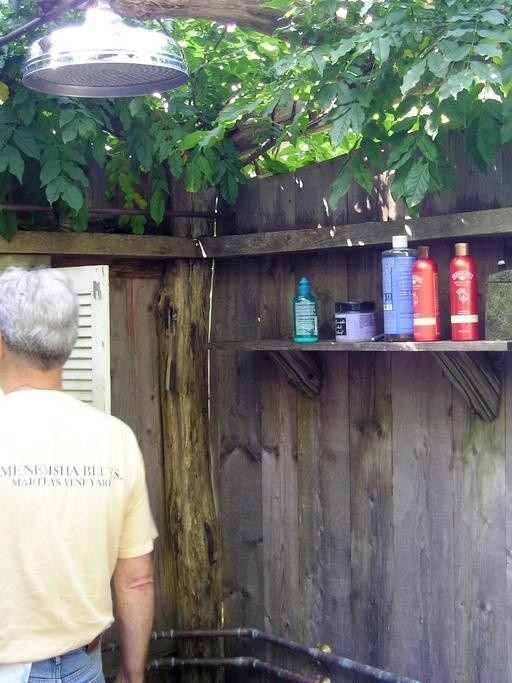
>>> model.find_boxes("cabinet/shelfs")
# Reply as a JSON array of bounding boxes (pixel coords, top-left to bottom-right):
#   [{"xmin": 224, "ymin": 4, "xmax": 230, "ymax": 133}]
[{"xmin": 201, "ymin": 332, "xmax": 509, "ymax": 416}]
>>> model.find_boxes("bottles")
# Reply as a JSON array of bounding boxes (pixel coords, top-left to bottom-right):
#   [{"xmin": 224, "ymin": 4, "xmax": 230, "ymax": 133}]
[{"xmin": 381, "ymin": 236, "xmax": 417, "ymax": 342}]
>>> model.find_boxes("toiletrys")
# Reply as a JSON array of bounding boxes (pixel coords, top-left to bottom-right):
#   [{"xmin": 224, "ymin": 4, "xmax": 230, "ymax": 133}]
[{"xmin": 378, "ymin": 234, "xmax": 412, "ymax": 340}]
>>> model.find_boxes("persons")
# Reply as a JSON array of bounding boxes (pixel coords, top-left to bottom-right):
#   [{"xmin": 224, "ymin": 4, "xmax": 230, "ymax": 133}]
[{"xmin": 0, "ymin": 264, "xmax": 160, "ymax": 683}]
